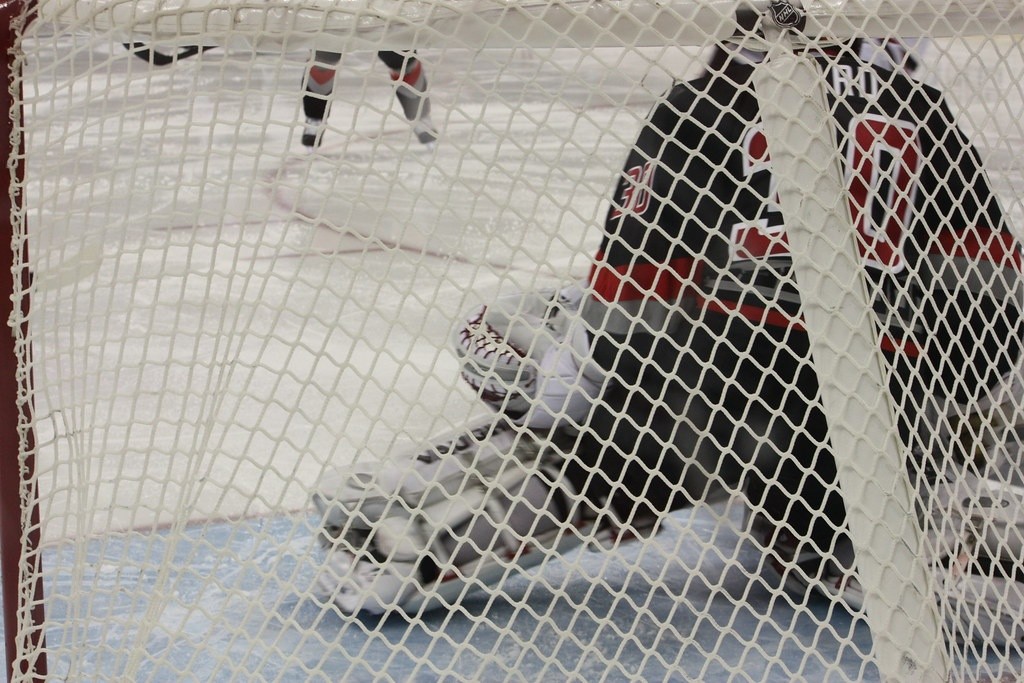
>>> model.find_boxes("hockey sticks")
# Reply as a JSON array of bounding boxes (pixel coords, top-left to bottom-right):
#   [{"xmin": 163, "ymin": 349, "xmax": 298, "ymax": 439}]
[{"xmin": 124, "ymin": 42, "xmax": 218, "ymax": 65}]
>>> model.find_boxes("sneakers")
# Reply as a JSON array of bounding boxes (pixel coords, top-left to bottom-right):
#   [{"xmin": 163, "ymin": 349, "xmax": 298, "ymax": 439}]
[
  {"xmin": 308, "ymin": 457, "xmax": 580, "ymax": 620},
  {"xmin": 301, "ymin": 118, "xmax": 326, "ymax": 150},
  {"xmin": 409, "ymin": 113, "xmax": 438, "ymax": 147}
]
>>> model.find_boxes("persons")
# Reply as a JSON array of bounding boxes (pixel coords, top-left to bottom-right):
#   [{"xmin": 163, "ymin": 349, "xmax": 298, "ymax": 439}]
[
  {"xmin": 312, "ymin": 46, "xmax": 1024, "ymax": 655},
  {"xmin": 299, "ymin": 48, "xmax": 439, "ymax": 150}
]
